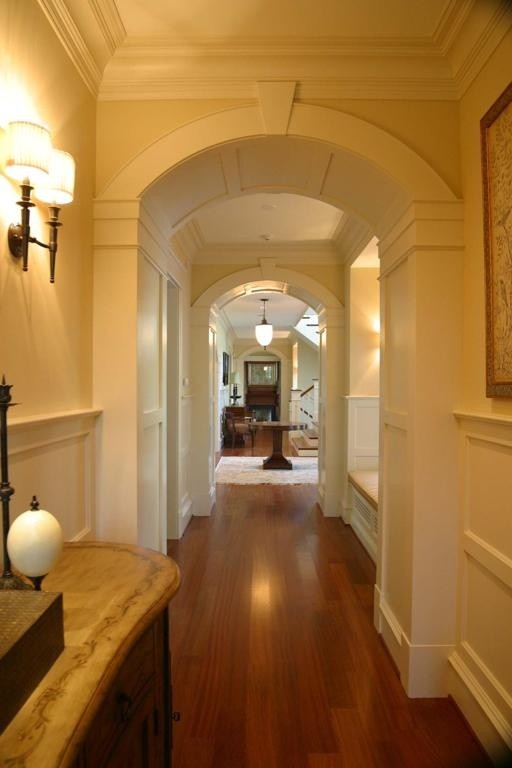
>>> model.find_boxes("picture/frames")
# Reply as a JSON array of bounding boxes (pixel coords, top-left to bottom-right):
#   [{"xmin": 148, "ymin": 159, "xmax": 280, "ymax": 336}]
[{"xmin": 479, "ymin": 76, "xmax": 512, "ymax": 400}]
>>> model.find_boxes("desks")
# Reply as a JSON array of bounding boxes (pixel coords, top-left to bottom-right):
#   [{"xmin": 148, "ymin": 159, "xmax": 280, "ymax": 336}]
[{"xmin": 248, "ymin": 421, "xmax": 308, "ymax": 470}]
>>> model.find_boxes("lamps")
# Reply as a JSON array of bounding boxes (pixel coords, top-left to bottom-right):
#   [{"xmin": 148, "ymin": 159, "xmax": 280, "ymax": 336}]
[
  {"xmin": 254, "ymin": 295, "xmax": 273, "ymax": 350},
  {"xmin": 4, "ymin": 111, "xmax": 79, "ymax": 287}
]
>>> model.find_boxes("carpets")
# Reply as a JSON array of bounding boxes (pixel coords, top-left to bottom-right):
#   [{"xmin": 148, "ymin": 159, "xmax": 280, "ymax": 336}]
[{"xmin": 214, "ymin": 453, "xmax": 320, "ymax": 485}]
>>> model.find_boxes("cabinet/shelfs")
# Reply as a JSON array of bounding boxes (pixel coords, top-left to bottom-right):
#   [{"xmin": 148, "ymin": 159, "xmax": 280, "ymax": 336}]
[{"xmin": 81, "ymin": 612, "xmax": 168, "ymax": 766}]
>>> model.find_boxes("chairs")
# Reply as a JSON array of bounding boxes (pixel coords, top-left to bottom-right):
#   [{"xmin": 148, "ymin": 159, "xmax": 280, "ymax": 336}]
[{"xmin": 221, "ymin": 406, "xmax": 257, "ymax": 450}]
